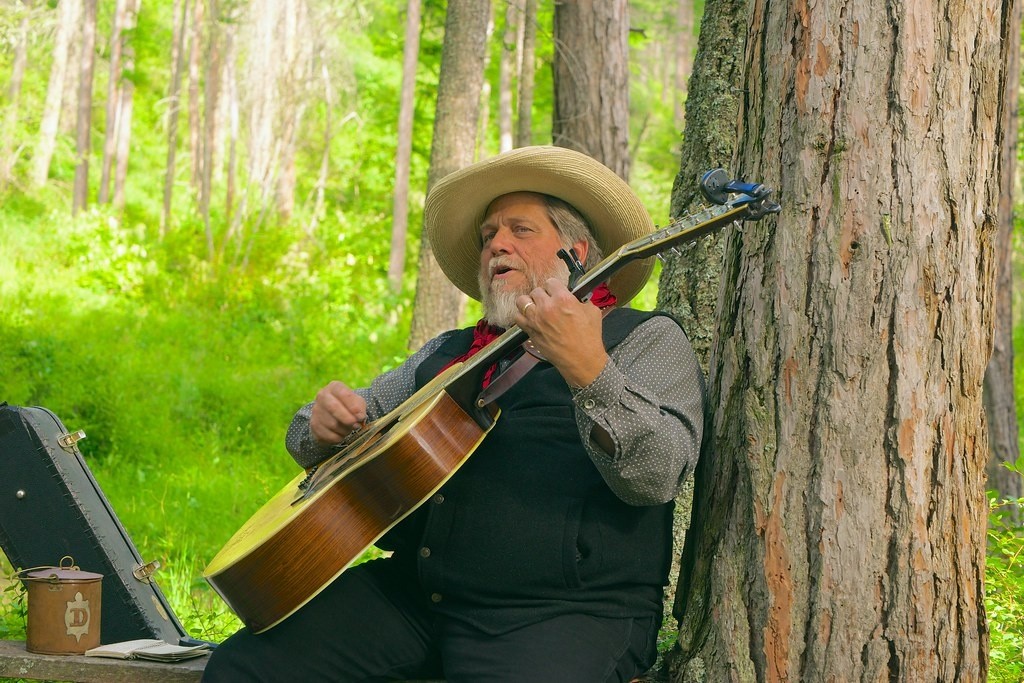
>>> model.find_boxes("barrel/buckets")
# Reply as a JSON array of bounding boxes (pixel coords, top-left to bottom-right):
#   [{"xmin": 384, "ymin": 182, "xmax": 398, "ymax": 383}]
[{"xmin": 13, "ymin": 556, "xmax": 104, "ymax": 656}]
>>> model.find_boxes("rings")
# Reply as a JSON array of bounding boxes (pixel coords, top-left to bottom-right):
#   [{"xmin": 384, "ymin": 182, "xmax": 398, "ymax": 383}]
[{"xmin": 521, "ymin": 299, "xmax": 535, "ymax": 315}]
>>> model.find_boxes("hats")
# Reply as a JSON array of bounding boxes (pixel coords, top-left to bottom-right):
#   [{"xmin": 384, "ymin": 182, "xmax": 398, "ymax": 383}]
[{"xmin": 424, "ymin": 146, "xmax": 656, "ymax": 308}]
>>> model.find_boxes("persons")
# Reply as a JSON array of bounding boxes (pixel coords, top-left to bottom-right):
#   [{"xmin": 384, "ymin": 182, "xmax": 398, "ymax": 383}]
[{"xmin": 202, "ymin": 144, "xmax": 704, "ymax": 682}]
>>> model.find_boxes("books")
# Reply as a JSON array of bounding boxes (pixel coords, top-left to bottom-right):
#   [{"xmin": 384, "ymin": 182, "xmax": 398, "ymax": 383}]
[{"xmin": 83, "ymin": 638, "xmax": 211, "ymax": 664}]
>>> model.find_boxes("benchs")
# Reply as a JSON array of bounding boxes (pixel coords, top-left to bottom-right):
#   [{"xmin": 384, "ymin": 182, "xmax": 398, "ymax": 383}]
[{"xmin": 0, "ymin": 639, "xmax": 212, "ymax": 683}]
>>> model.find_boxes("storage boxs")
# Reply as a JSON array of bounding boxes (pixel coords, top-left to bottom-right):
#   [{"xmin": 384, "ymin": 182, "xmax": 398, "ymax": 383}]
[
  {"xmin": 0, "ymin": 398, "xmax": 195, "ymax": 645},
  {"xmin": 24, "ymin": 555, "xmax": 105, "ymax": 655}
]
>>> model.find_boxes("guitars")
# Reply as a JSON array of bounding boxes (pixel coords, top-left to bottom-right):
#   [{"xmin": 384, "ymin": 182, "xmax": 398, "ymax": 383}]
[{"xmin": 202, "ymin": 163, "xmax": 783, "ymax": 637}]
